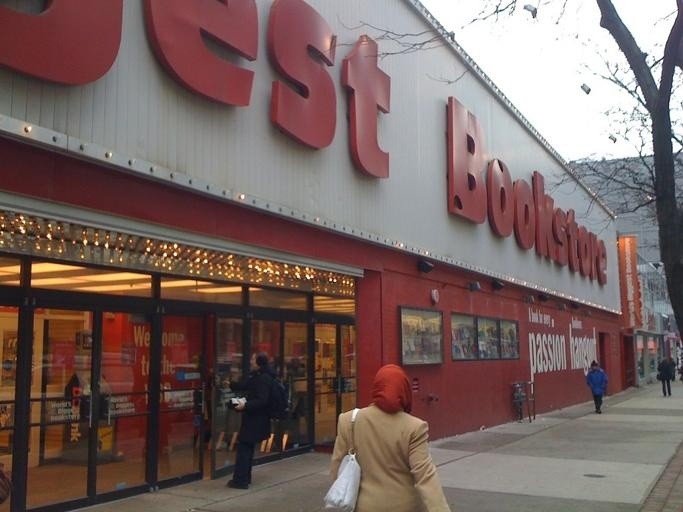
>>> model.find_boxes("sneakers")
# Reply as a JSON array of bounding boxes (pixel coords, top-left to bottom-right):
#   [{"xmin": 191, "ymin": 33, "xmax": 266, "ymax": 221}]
[{"xmin": 227, "ymin": 480, "xmax": 252, "ymax": 489}]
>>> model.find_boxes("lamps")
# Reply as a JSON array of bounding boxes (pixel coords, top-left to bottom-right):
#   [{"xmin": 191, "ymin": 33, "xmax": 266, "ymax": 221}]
[{"xmin": 417, "ymin": 259, "xmax": 593, "ymax": 318}]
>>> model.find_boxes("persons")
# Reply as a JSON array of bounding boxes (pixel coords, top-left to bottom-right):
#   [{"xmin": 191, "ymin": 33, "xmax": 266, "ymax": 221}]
[
  {"xmin": 328, "ymin": 365, "xmax": 450, "ymax": 512},
  {"xmin": 586, "ymin": 359, "xmax": 608, "ymax": 414},
  {"xmin": 678, "ymin": 364, "xmax": 683, "ymax": 382},
  {"xmin": 222, "ymin": 352, "xmax": 284, "ymax": 488},
  {"xmin": 656, "ymin": 358, "xmax": 671, "ymax": 396},
  {"xmin": 669, "ymin": 358, "xmax": 677, "ymax": 381}
]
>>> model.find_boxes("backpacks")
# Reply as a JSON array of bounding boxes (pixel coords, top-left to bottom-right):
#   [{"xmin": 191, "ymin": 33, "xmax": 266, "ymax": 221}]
[{"xmin": 266, "ymin": 376, "xmax": 292, "ymax": 421}]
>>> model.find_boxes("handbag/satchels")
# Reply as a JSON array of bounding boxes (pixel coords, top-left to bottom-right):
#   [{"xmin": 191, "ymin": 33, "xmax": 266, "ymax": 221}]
[{"xmin": 323, "ymin": 448, "xmax": 361, "ymax": 512}]
